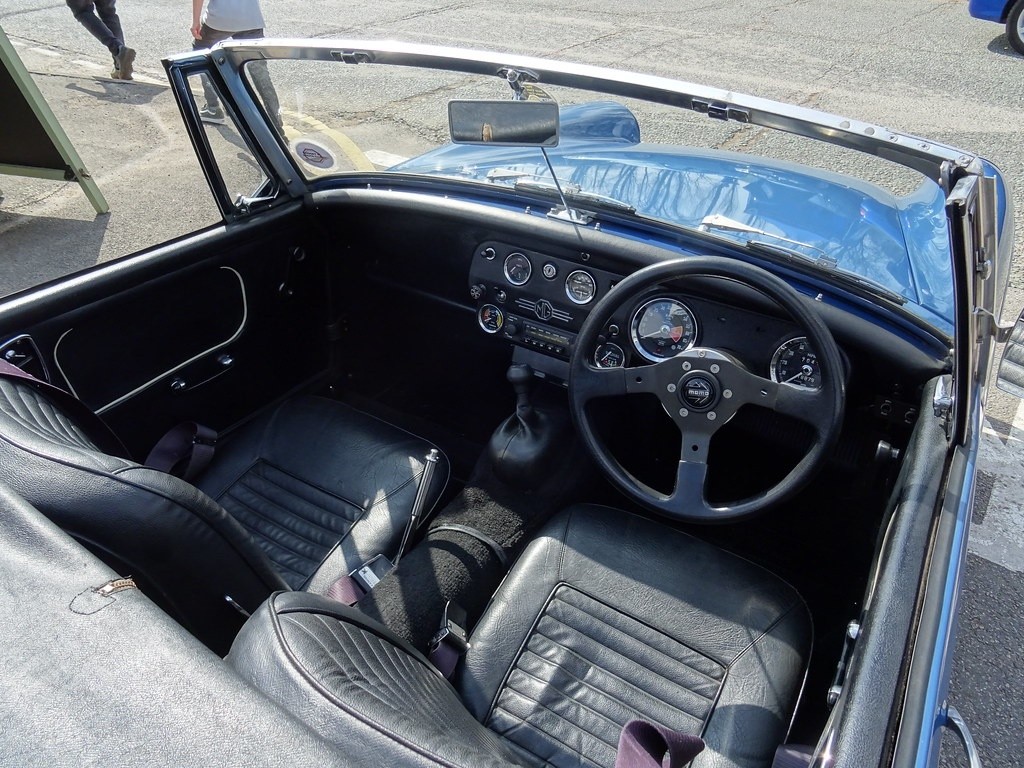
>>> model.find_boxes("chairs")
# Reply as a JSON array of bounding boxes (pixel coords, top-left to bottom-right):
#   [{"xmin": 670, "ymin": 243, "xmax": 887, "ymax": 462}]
[
  {"xmin": 0, "ymin": 355, "xmax": 461, "ymax": 648},
  {"xmin": 221, "ymin": 502, "xmax": 814, "ymax": 768}
]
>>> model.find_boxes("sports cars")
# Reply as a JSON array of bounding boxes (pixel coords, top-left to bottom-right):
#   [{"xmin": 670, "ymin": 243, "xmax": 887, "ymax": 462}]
[{"xmin": 0, "ymin": 30, "xmax": 1024, "ymax": 768}]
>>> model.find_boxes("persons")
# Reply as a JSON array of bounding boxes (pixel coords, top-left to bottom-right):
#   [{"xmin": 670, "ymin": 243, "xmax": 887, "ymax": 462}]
[
  {"xmin": 190, "ymin": 0, "xmax": 283, "ymax": 128},
  {"xmin": 66, "ymin": 0, "xmax": 136, "ymax": 80}
]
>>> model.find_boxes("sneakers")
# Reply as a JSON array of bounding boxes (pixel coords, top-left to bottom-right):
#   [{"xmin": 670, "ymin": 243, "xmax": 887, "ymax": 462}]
[
  {"xmin": 110, "ymin": 46, "xmax": 136, "ymax": 80},
  {"xmin": 196, "ymin": 105, "xmax": 225, "ymax": 125}
]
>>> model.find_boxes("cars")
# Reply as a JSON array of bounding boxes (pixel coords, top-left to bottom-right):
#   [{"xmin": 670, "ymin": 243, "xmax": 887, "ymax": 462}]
[{"xmin": 968, "ymin": 0, "xmax": 1024, "ymax": 57}]
